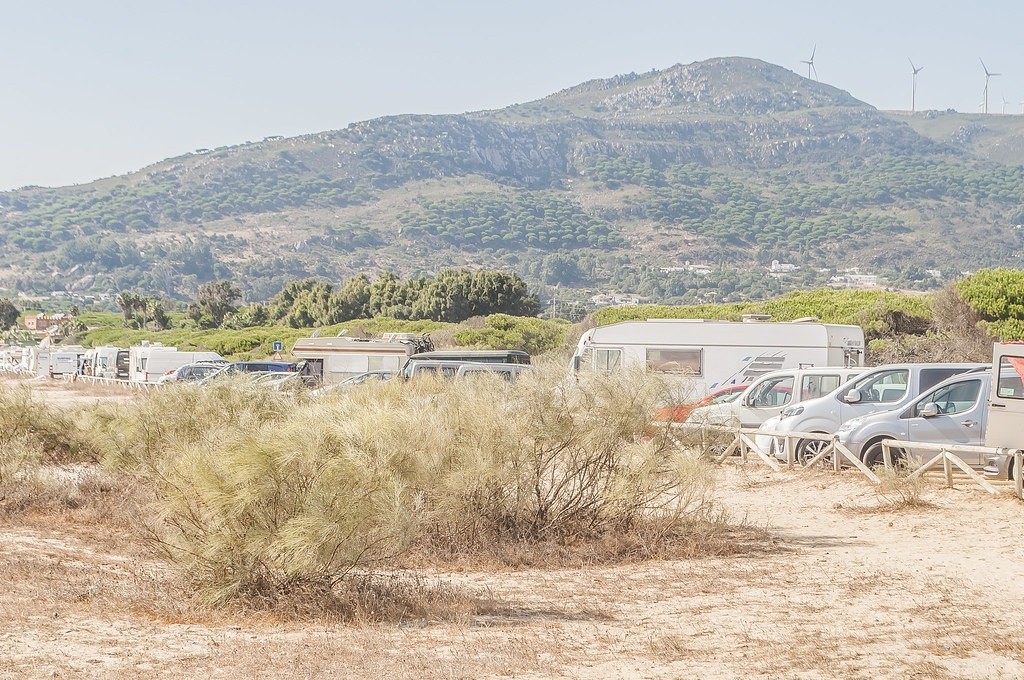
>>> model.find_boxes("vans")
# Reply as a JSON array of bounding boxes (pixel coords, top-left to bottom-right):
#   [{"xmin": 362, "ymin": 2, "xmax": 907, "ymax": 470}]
[
  {"xmin": 391, "ymin": 350, "xmax": 537, "ymax": 385},
  {"xmin": 199, "ymin": 362, "xmax": 297, "ymax": 386},
  {"xmin": 555, "ymin": 314, "xmax": 864, "ymax": 421},
  {"xmin": 140, "ymin": 349, "xmax": 226, "ymax": 384},
  {"xmin": 774, "ymin": 363, "xmax": 1003, "ymax": 471}
]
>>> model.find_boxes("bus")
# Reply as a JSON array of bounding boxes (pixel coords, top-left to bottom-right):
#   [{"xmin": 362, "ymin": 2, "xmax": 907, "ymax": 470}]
[{"xmin": 684, "ymin": 362, "xmax": 897, "ymax": 457}]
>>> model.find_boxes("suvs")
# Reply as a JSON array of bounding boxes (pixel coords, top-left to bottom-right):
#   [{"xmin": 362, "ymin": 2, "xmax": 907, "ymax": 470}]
[
  {"xmin": 173, "ymin": 360, "xmax": 229, "ymax": 382},
  {"xmin": 831, "ymin": 364, "xmax": 1024, "ymax": 484}
]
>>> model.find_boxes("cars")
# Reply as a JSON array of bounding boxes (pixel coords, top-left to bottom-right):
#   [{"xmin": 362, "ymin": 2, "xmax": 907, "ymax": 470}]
[
  {"xmin": 656, "ymin": 382, "xmax": 807, "ymax": 424},
  {"xmin": 755, "ymin": 384, "xmax": 908, "ymax": 464},
  {"xmin": 229, "ymin": 369, "xmax": 400, "ymax": 395}
]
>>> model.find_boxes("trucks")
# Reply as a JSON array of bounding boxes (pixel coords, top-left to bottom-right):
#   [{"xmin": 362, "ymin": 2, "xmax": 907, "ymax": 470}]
[{"xmin": 23, "ymin": 342, "xmax": 176, "ymax": 383}]
